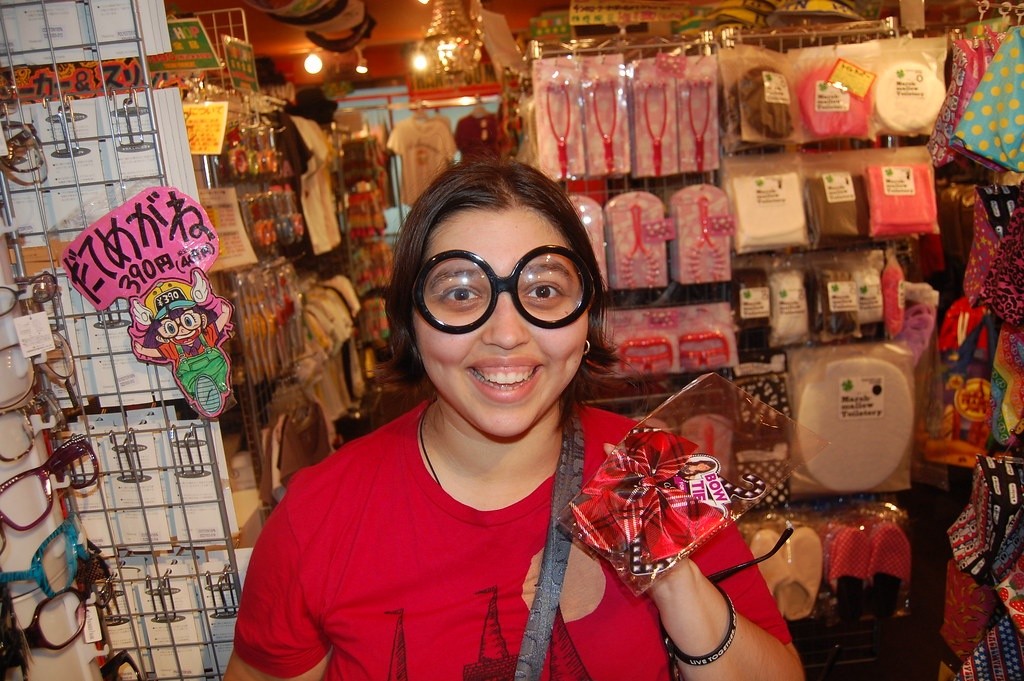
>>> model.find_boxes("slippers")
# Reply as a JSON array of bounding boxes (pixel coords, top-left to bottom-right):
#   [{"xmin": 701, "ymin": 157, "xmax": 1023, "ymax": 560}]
[{"xmin": 750, "ymin": 507, "xmax": 919, "ymax": 630}]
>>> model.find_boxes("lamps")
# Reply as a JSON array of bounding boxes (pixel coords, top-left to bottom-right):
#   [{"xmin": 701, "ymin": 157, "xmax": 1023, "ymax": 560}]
[{"xmin": 354, "ymin": 44, "xmax": 368, "ymax": 74}]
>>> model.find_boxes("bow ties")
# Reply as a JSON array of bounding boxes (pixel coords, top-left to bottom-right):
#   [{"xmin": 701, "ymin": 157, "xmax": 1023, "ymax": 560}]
[{"xmin": 571, "ymin": 426, "xmax": 726, "ymax": 564}]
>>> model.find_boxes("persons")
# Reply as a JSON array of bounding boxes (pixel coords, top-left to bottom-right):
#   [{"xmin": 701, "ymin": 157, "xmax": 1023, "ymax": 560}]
[{"xmin": 222, "ymin": 160, "xmax": 807, "ymax": 681}]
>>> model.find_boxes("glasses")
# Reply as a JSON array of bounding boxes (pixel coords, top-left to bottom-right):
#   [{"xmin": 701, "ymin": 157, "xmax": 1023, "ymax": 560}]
[
  {"xmin": 0, "ymin": 119, "xmax": 143, "ymax": 681},
  {"xmin": 411, "ymin": 244, "xmax": 594, "ymax": 335}
]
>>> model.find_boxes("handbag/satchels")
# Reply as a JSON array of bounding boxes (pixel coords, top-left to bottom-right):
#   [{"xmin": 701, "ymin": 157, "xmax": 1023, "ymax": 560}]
[{"xmin": 921, "ymin": 315, "xmax": 1002, "ymax": 471}]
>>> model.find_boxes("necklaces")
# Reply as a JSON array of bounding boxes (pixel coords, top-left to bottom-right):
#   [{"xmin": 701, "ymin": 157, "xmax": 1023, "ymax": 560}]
[{"xmin": 419, "ymin": 398, "xmax": 444, "ymax": 488}]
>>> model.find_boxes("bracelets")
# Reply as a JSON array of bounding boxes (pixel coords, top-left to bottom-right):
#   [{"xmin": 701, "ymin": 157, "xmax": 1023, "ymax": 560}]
[{"xmin": 660, "ymin": 583, "xmax": 738, "ymax": 666}]
